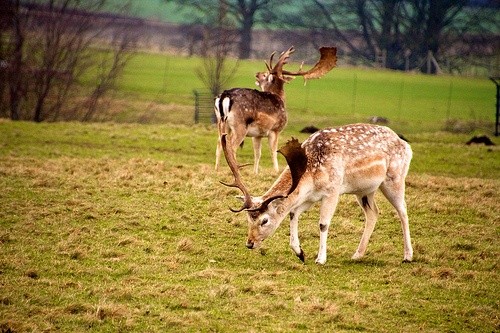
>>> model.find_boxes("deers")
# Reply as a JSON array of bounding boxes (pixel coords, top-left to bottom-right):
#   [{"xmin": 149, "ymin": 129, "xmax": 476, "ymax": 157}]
[
  {"xmin": 216, "ymin": 123, "xmax": 414, "ymax": 266},
  {"xmin": 213, "ymin": 42, "xmax": 338, "ymax": 176}
]
